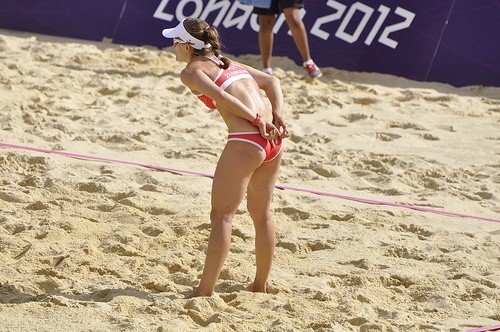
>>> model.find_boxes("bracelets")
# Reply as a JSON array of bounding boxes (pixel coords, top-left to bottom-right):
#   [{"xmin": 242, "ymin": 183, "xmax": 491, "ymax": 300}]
[{"xmin": 251, "ymin": 113, "xmax": 261, "ymax": 128}]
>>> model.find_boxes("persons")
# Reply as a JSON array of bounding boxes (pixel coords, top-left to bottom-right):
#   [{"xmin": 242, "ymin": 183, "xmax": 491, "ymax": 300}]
[
  {"xmin": 252, "ymin": 0, "xmax": 323, "ymax": 83},
  {"xmin": 162, "ymin": 16, "xmax": 290, "ymax": 301}
]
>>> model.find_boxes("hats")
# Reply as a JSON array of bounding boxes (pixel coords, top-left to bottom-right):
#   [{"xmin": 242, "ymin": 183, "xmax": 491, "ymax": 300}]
[{"xmin": 162, "ymin": 19, "xmax": 211, "ymax": 50}]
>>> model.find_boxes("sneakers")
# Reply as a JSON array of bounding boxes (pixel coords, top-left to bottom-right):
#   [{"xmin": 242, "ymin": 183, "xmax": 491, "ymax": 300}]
[{"xmin": 305, "ymin": 64, "xmax": 322, "ymax": 79}]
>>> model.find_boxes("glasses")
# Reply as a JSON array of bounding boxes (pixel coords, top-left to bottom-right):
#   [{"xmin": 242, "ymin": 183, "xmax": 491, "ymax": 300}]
[{"xmin": 172, "ymin": 38, "xmax": 195, "ymax": 45}]
